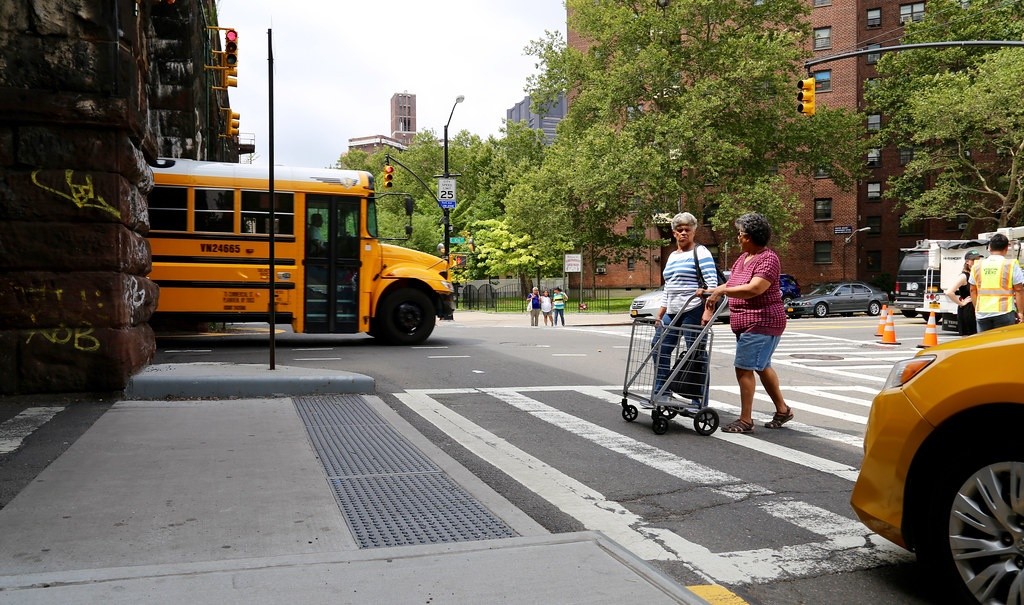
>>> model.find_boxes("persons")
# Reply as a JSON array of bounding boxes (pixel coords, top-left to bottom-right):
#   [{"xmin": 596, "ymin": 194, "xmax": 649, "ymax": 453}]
[
  {"xmin": 526, "ymin": 287, "xmax": 541, "ymax": 326},
  {"xmin": 695, "ymin": 213, "xmax": 794, "ymax": 433},
  {"xmin": 945, "ymin": 250, "xmax": 984, "ymax": 337},
  {"xmin": 968, "ymin": 233, "xmax": 1024, "ymax": 334},
  {"xmin": 309, "ymin": 214, "xmax": 326, "ymax": 256},
  {"xmin": 540, "ymin": 290, "xmax": 553, "ymax": 326},
  {"xmin": 551, "ymin": 286, "xmax": 568, "ymax": 327},
  {"xmin": 640, "ymin": 210, "xmax": 717, "ymax": 417}
]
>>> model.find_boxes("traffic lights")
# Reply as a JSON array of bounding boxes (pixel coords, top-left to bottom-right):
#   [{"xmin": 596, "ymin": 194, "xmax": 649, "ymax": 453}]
[
  {"xmin": 383, "ymin": 165, "xmax": 394, "ymax": 189},
  {"xmin": 797, "ymin": 77, "xmax": 815, "ymax": 115},
  {"xmin": 224, "ymin": 30, "xmax": 239, "ymax": 66},
  {"xmin": 457, "ymin": 255, "xmax": 466, "ymax": 269},
  {"xmin": 222, "ymin": 53, "xmax": 238, "ymax": 88},
  {"xmin": 228, "ymin": 109, "xmax": 240, "ymax": 136}
]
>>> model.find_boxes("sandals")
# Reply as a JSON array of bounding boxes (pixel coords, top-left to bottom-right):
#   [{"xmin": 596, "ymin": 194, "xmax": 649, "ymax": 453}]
[
  {"xmin": 721, "ymin": 419, "xmax": 755, "ymax": 433},
  {"xmin": 765, "ymin": 405, "xmax": 794, "ymax": 428}
]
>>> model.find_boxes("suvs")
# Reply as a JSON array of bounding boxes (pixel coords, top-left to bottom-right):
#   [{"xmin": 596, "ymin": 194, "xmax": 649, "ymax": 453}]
[{"xmin": 895, "ymin": 239, "xmax": 941, "ymax": 322}]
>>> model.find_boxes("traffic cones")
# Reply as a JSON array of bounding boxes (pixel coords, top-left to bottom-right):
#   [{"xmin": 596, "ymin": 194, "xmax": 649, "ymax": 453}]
[
  {"xmin": 915, "ymin": 311, "xmax": 938, "ymax": 349},
  {"xmin": 876, "ymin": 309, "xmax": 902, "ymax": 345},
  {"xmin": 874, "ymin": 305, "xmax": 888, "ymax": 336}
]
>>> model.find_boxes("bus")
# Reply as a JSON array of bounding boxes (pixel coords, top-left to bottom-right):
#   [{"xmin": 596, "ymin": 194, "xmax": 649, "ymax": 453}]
[{"xmin": 148, "ymin": 158, "xmax": 481, "ymax": 346}]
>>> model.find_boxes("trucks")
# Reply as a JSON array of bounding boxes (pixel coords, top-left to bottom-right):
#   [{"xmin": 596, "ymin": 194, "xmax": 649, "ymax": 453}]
[{"xmin": 915, "ymin": 226, "xmax": 1024, "ymax": 331}]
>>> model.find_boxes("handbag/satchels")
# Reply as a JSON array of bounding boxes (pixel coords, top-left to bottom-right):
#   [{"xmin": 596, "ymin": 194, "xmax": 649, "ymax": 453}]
[
  {"xmin": 666, "ymin": 351, "xmax": 704, "ymax": 400},
  {"xmin": 527, "ymin": 302, "xmax": 532, "ymax": 311},
  {"xmin": 694, "ymin": 244, "xmax": 730, "ymax": 312}
]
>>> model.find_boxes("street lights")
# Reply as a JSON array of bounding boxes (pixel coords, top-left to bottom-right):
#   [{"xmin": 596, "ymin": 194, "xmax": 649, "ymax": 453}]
[{"xmin": 440, "ymin": 94, "xmax": 465, "ymax": 321}]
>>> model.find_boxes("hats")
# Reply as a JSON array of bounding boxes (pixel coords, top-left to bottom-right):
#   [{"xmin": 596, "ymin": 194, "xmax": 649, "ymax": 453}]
[{"xmin": 964, "ymin": 250, "xmax": 984, "ymax": 260}]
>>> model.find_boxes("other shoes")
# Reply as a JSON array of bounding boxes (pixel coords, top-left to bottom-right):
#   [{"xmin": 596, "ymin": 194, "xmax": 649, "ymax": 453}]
[
  {"xmin": 640, "ymin": 400, "xmax": 664, "ymax": 409},
  {"xmin": 684, "ymin": 408, "xmax": 700, "ymax": 414}
]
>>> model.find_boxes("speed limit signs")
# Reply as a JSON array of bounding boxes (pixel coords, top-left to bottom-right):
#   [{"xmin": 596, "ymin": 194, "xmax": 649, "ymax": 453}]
[{"xmin": 439, "ymin": 178, "xmax": 456, "ymax": 200}]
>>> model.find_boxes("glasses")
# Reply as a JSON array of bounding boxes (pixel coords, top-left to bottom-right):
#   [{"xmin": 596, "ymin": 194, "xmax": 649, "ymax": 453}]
[{"xmin": 676, "ymin": 226, "xmax": 692, "ymax": 233}]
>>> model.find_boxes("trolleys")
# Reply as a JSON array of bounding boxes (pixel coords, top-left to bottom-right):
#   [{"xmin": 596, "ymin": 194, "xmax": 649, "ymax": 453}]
[{"xmin": 622, "ymin": 292, "xmax": 728, "ymax": 436}]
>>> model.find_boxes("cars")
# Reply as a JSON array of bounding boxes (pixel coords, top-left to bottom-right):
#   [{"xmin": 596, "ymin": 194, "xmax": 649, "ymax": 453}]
[
  {"xmin": 629, "ymin": 272, "xmax": 801, "ymax": 324},
  {"xmin": 783, "ymin": 282, "xmax": 889, "ymax": 318},
  {"xmin": 850, "ymin": 321, "xmax": 1024, "ymax": 605}
]
